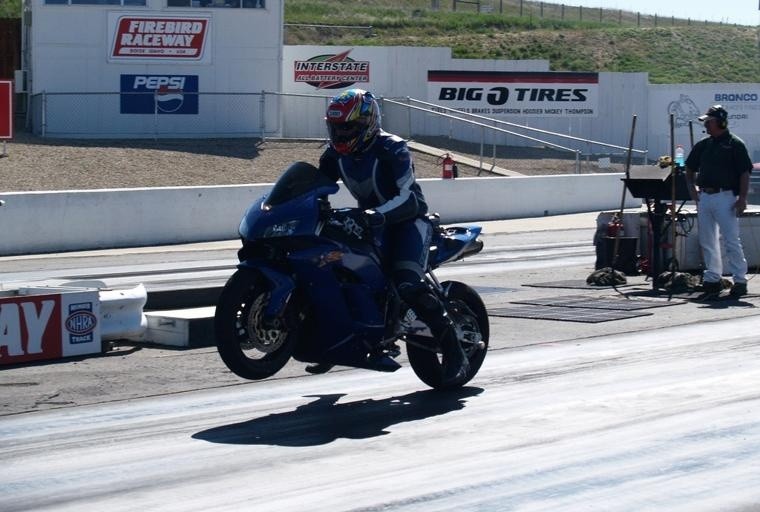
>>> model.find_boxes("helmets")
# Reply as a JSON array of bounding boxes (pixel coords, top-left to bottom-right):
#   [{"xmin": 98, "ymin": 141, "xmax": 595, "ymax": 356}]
[{"xmin": 325, "ymin": 88, "xmax": 381, "ymax": 159}]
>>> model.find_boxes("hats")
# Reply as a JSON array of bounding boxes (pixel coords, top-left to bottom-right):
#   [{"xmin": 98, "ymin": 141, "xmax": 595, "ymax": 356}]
[{"xmin": 698, "ymin": 104, "xmax": 728, "ymax": 122}]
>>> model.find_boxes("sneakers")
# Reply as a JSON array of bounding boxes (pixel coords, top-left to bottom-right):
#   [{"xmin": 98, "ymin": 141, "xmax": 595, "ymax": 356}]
[
  {"xmin": 696, "ymin": 281, "xmax": 722, "ymax": 292},
  {"xmin": 730, "ymin": 282, "xmax": 747, "ymax": 297}
]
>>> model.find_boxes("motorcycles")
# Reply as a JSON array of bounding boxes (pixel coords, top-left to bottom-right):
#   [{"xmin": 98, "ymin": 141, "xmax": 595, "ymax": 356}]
[{"xmin": 216, "ymin": 162, "xmax": 489, "ymax": 389}]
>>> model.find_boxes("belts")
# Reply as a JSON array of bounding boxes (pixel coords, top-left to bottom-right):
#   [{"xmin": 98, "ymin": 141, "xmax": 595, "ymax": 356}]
[{"xmin": 699, "ymin": 186, "xmax": 733, "ymax": 194}]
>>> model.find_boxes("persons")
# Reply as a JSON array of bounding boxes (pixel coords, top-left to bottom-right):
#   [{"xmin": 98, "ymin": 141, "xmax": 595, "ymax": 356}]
[
  {"xmin": 305, "ymin": 89, "xmax": 473, "ymax": 386},
  {"xmin": 683, "ymin": 107, "xmax": 752, "ymax": 300}
]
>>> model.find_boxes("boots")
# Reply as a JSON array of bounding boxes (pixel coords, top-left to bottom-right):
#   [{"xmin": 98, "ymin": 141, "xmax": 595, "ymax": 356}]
[{"xmin": 399, "ymin": 281, "xmax": 470, "ymax": 386}]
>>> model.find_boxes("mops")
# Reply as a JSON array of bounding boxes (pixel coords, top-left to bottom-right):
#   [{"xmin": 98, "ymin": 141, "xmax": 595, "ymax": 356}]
[{"xmin": 585, "ymin": 114, "xmax": 639, "ymax": 287}]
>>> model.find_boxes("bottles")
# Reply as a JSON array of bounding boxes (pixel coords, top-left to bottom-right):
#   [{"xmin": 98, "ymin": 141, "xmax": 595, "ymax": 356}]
[{"xmin": 674, "ymin": 144, "xmax": 684, "ymax": 167}]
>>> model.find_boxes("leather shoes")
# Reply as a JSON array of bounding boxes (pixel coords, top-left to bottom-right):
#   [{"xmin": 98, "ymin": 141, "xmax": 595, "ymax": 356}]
[{"xmin": 305, "ymin": 361, "xmax": 335, "ymax": 374}]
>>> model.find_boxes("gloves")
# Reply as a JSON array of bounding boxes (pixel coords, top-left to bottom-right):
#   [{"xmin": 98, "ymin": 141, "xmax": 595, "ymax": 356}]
[{"xmin": 330, "ymin": 207, "xmax": 385, "ymax": 239}]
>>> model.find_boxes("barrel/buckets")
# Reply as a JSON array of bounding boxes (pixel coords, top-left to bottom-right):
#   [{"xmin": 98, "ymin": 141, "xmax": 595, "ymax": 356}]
[
  {"xmin": 592, "ymin": 212, "xmax": 640, "ymax": 274},
  {"xmin": 674, "ymin": 214, "xmax": 705, "ymax": 271},
  {"xmin": 736, "ymin": 214, "xmax": 760, "ymax": 271}
]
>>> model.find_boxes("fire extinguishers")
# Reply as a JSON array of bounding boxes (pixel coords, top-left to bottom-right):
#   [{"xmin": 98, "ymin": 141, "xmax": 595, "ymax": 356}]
[{"xmin": 436, "ymin": 153, "xmax": 453, "ymax": 179}]
